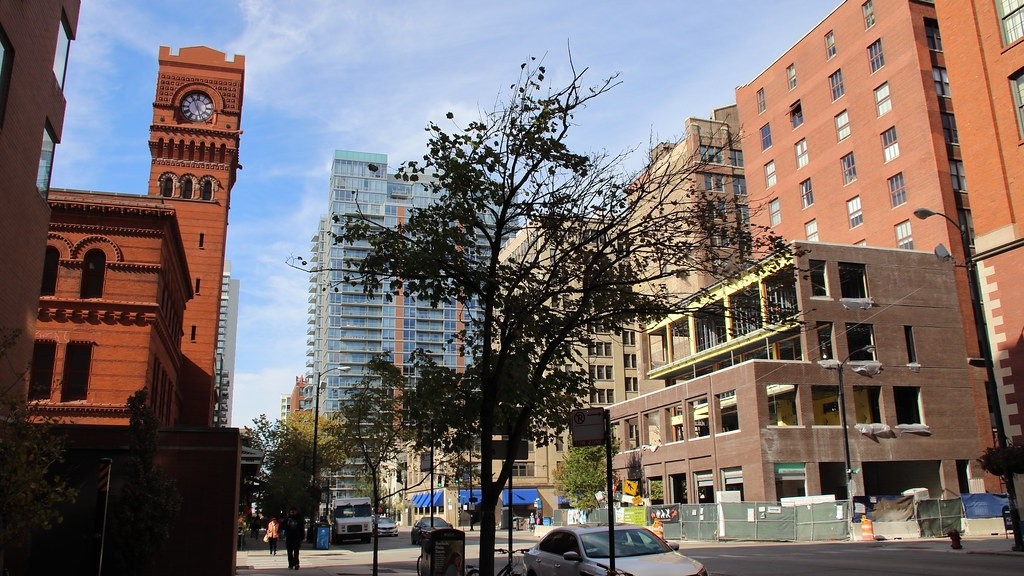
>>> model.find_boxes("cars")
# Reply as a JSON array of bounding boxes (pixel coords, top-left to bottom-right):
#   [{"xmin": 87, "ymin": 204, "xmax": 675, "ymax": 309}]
[
  {"xmin": 523, "ymin": 521, "xmax": 709, "ymax": 576},
  {"xmin": 372, "ymin": 516, "xmax": 399, "ymax": 537},
  {"xmin": 410, "ymin": 517, "xmax": 454, "ymax": 546},
  {"xmin": 499, "ymin": 516, "xmax": 524, "ymax": 530}
]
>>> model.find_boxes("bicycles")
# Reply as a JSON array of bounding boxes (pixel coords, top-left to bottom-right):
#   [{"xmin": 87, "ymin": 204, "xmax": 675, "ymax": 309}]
[{"xmin": 465, "ymin": 547, "xmax": 530, "ymax": 576}]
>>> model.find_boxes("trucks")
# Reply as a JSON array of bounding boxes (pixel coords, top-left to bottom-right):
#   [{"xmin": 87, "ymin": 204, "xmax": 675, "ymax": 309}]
[{"xmin": 331, "ymin": 497, "xmax": 373, "ymax": 544}]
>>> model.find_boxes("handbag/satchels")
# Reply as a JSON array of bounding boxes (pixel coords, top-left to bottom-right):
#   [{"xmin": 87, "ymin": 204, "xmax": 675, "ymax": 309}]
[{"xmin": 263, "ymin": 533, "xmax": 269, "ymax": 543}]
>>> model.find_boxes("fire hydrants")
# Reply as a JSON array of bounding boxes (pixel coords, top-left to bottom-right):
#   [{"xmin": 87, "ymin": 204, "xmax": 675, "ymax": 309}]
[{"xmin": 946, "ymin": 528, "xmax": 964, "ymax": 549}]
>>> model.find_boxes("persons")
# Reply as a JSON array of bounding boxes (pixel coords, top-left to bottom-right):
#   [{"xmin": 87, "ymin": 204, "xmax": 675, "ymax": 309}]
[
  {"xmin": 252, "ymin": 515, "xmax": 262, "ymax": 540},
  {"xmin": 283, "ymin": 508, "xmax": 306, "ymax": 570},
  {"xmin": 264, "ymin": 517, "xmax": 281, "ymax": 556},
  {"xmin": 529, "ymin": 512, "xmax": 536, "ymax": 531}
]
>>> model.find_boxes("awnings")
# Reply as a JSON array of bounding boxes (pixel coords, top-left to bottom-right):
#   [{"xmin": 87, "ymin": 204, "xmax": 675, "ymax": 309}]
[
  {"xmin": 409, "ymin": 490, "xmax": 444, "ymax": 507},
  {"xmin": 502, "ymin": 489, "xmax": 538, "ymax": 505},
  {"xmin": 460, "ymin": 489, "xmax": 482, "ymax": 504}
]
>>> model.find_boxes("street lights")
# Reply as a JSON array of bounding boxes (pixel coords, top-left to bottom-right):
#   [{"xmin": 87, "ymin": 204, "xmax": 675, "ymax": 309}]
[
  {"xmin": 307, "ymin": 366, "xmax": 353, "ymax": 540},
  {"xmin": 913, "ymin": 207, "xmax": 1023, "ymax": 551},
  {"xmin": 837, "ymin": 344, "xmax": 878, "ymax": 500}
]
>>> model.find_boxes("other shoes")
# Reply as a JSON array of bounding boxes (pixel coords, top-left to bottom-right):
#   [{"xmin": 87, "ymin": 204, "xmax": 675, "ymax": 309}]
[
  {"xmin": 294, "ymin": 564, "xmax": 301, "ymax": 570},
  {"xmin": 270, "ymin": 550, "xmax": 272, "ymax": 554},
  {"xmin": 274, "ymin": 551, "xmax": 276, "ymax": 555}
]
